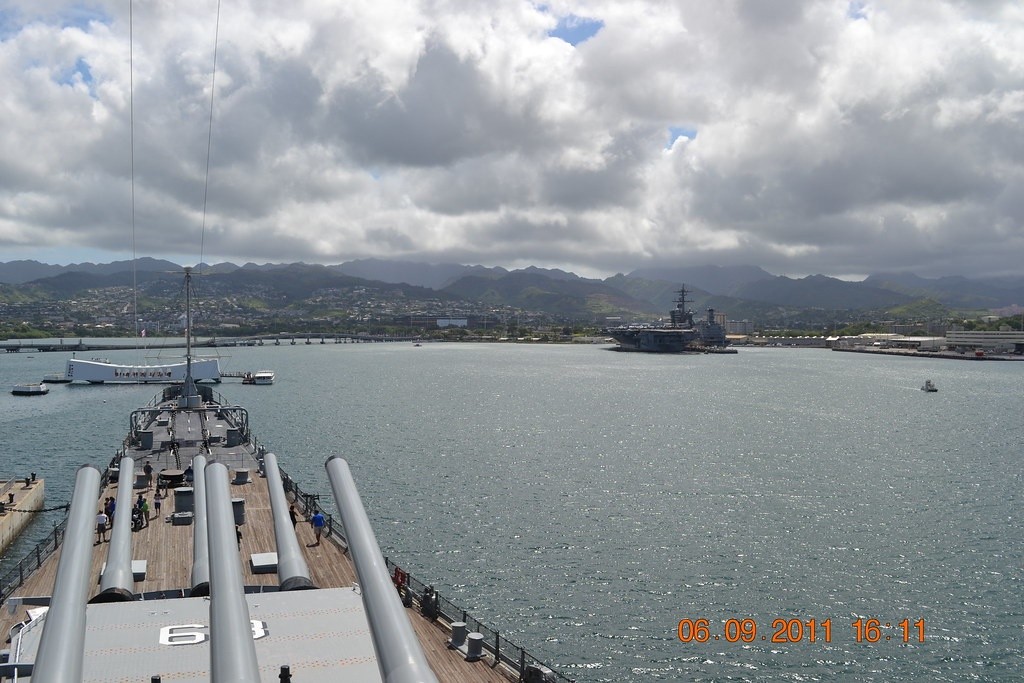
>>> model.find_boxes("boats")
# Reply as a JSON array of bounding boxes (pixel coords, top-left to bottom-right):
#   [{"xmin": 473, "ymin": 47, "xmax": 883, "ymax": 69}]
[
  {"xmin": 254, "ymin": 370, "xmax": 276, "ymax": 385},
  {"xmin": 41, "ymin": 371, "xmax": 72, "ymax": 383},
  {"xmin": 921, "ymin": 379, "xmax": 938, "ymax": 392},
  {"xmin": 414, "ymin": 343, "xmax": 422, "ymax": 347},
  {"xmin": 10, "ymin": 381, "xmax": 49, "ymax": 395}
]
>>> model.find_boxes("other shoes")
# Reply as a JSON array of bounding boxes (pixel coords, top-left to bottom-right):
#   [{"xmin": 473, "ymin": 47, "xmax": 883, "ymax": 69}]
[
  {"xmin": 104, "ymin": 540, "xmax": 106, "ymax": 542},
  {"xmin": 315, "ymin": 542, "xmax": 320, "ymax": 545},
  {"xmin": 146, "ymin": 524, "xmax": 148, "ymax": 526},
  {"xmin": 96, "ymin": 541, "xmax": 100, "ymax": 544}
]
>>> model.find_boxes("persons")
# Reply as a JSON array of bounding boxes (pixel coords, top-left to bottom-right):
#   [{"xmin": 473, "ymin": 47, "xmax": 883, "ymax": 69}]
[
  {"xmin": 143, "ymin": 460, "xmax": 155, "ymax": 490},
  {"xmin": 183, "ymin": 465, "xmax": 193, "ymax": 486},
  {"xmin": 131, "ymin": 495, "xmax": 150, "ymax": 532},
  {"xmin": 96, "ymin": 496, "xmax": 116, "ymax": 544},
  {"xmin": 235, "ymin": 525, "xmax": 243, "ymax": 551},
  {"xmin": 153, "ymin": 488, "xmax": 163, "ymax": 518},
  {"xmin": 311, "ymin": 509, "xmax": 326, "ymax": 546},
  {"xmin": 289, "ymin": 505, "xmax": 298, "ymax": 530}
]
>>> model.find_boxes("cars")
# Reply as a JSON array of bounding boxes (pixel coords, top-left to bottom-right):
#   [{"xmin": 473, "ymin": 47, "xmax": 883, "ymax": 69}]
[
  {"xmin": 879, "ymin": 346, "xmax": 889, "ymax": 349},
  {"xmin": 854, "ymin": 342, "xmax": 860, "ymax": 345},
  {"xmin": 940, "ymin": 346, "xmax": 948, "ymax": 350},
  {"xmin": 975, "ymin": 347, "xmax": 984, "ymax": 356}
]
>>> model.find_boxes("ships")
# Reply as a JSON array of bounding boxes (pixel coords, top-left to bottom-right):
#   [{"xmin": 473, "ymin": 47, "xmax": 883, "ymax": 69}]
[
  {"xmin": 606, "ymin": 283, "xmax": 700, "ymax": 352},
  {"xmin": 0, "ymin": 265, "xmax": 587, "ymax": 683},
  {"xmin": 695, "ymin": 307, "xmax": 731, "ymax": 348}
]
